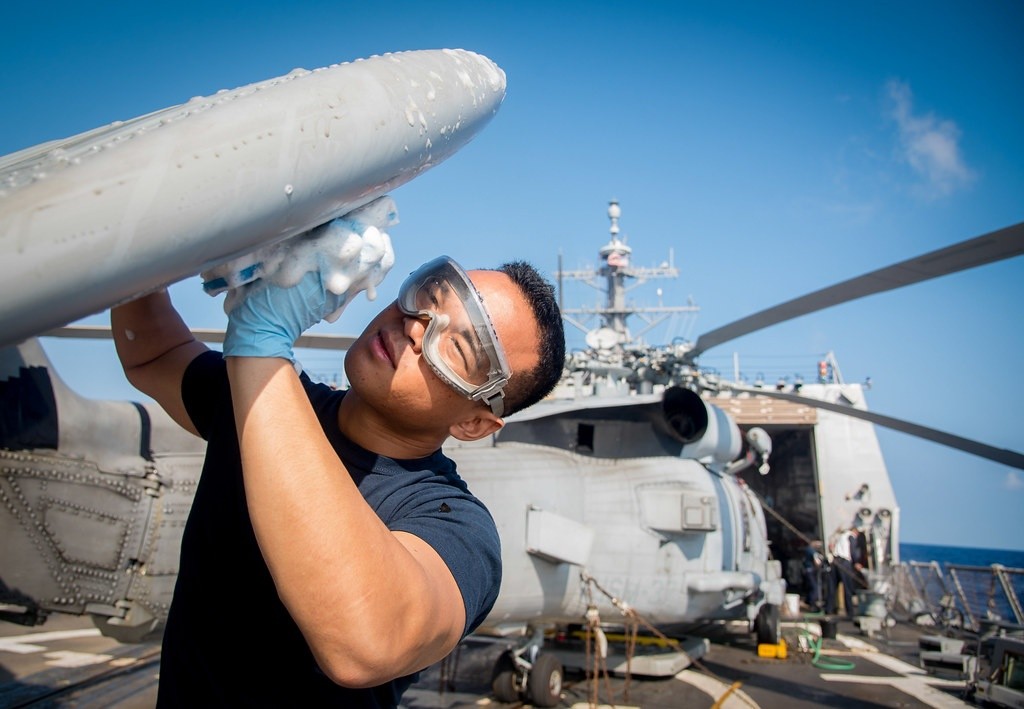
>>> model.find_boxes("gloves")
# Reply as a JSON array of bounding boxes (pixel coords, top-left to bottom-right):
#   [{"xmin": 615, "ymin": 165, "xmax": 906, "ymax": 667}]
[{"xmin": 222, "ymin": 218, "xmax": 395, "ymax": 363}]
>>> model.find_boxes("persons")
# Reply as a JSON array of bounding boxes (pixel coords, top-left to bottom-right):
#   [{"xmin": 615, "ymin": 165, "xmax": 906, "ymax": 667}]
[
  {"xmin": 785, "ymin": 526, "xmax": 863, "ymax": 616},
  {"xmin": 108, "ymin": 218, "xmax": 568, "ymax": 709}
]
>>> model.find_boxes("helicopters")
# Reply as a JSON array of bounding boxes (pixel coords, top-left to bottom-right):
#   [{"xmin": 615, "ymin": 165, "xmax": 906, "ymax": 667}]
[{"xmin": 0, "ymin": 176, "xmax": 1024, "ymax": 709}]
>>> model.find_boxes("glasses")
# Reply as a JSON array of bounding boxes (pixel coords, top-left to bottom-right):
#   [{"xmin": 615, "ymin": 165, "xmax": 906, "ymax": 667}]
[{"xmin": 398, "ymin": 254, "xmax": 513, "ymax": 406}]
